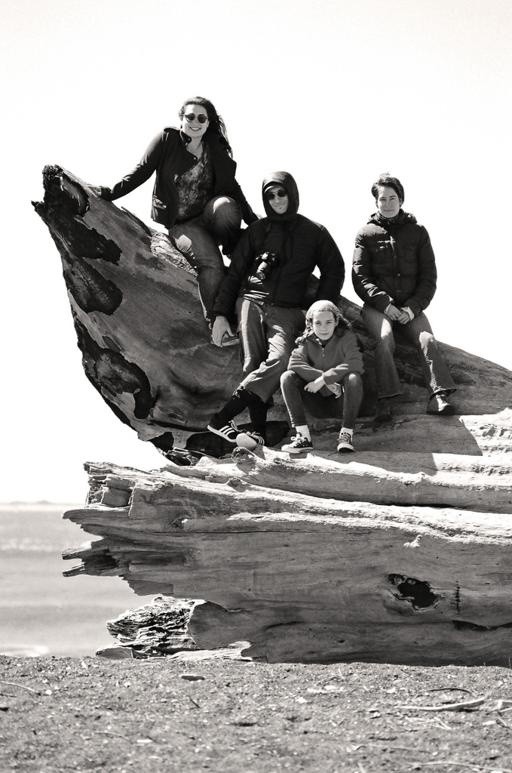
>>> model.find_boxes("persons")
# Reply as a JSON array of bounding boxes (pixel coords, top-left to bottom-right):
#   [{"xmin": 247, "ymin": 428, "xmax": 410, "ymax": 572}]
[
  {"xmin": 207, "ymin": 170, "xmax": 345, "ymax": 444},
  {"xmin": 85, "ymin": 95, "xmax": 258, "ymax": 349},
  {"xmin": 279, "ymin": 299, "xmax": 364, "ymax": 454},
  {"xmin": 351, "ymin": 173, "xmax": 457, "ymax": 421}
]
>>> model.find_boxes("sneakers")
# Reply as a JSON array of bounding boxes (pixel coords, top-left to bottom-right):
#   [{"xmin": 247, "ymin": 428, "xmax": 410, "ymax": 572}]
[
  {"xmin": 426, "ymin": 392, "xmax": 452, "ymax": 415},
  {"xmin": 374, "ymin": 403, "xmax": 393, "ymax": 422},
  {"xmin": 207, "ymin": 330, "xmax": 241, "ymax": 347},
  {"xmin": 235, "ymin": 430, "xmax": 268, "ymax": 451},
  {"xmin": 335, "ymin": 431, "xmax": 354, "ymax": 452},
  {"xmin": 281, "ymin": 433, "xmax": 314, "ymax": 454},
  {"xmin": 207, "ymin": 411, "xmax": 251, "ymax": 442}
]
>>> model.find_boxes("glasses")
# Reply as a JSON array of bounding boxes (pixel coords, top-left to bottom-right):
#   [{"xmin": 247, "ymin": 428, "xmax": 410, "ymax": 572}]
[
  {"xmin": 182, "ymin": 112, "xmax": 209, "ymax": 124},
  {"xmin": 265, "ymin": 188, "xmax": 288, "ymax": 200}
]
